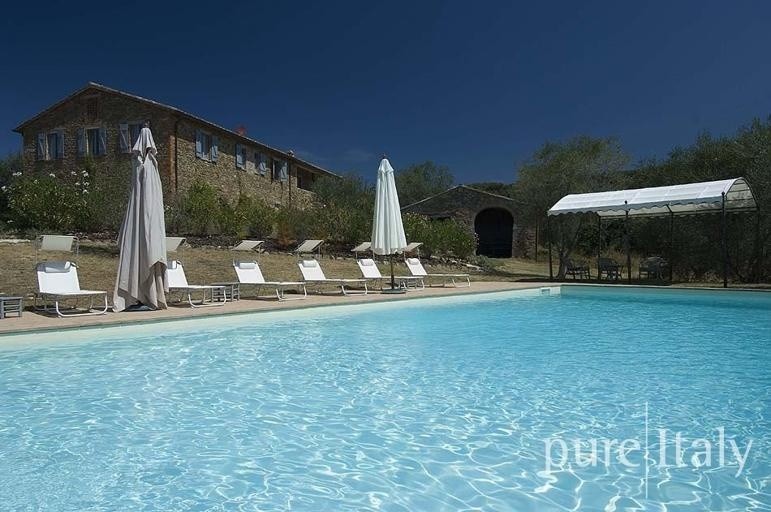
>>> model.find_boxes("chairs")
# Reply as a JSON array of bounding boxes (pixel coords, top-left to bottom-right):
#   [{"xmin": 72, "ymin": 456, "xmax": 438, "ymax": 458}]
[
  {"xmin": 31, "ymin": 235, "xmax": 471, "ymax": 318},
  {"xmin": 561, "ymin": 256, "xmax": 661, "ymax": 283}
]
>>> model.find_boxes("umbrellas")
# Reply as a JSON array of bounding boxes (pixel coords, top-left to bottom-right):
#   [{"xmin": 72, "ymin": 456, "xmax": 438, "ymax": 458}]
[
  {"xmin": 369, "ymin": 156, "xmax": 409, "ymax": 287},
  {"xmin": 109, "ymin": 115, "xmax": 169, "ymax": 315}
]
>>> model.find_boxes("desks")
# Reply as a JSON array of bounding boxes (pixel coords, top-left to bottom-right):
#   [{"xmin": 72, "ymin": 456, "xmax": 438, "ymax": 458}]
[{"xmin": 0, "ymin": 296, "xmax": 24, "ymax": 319}]
